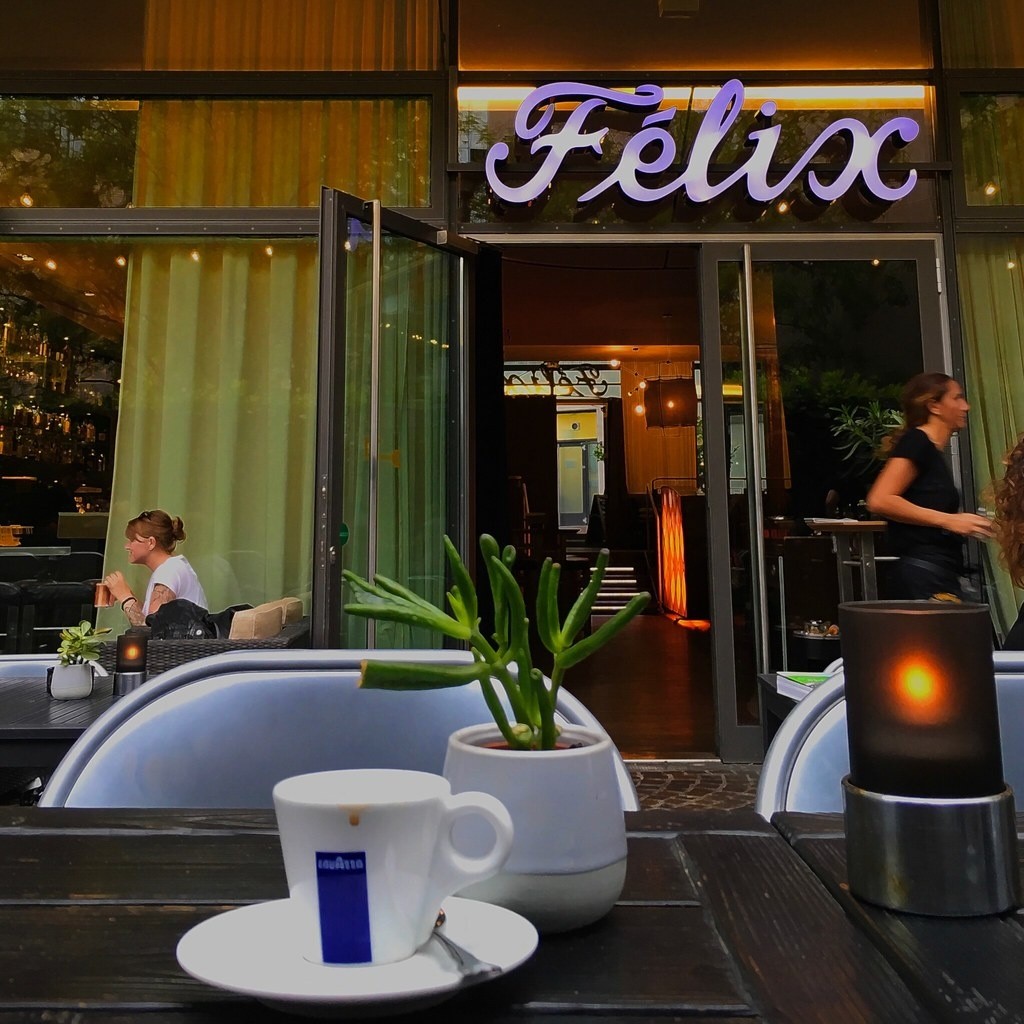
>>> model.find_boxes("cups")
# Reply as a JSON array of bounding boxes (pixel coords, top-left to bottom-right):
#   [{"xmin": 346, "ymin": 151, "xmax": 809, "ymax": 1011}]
[
  {"xmin": 94, "ymin": 583, "xmax": 115, "ymax": 607},
  {"xmin": 271, "ymin": 768, "xmax": 516, "ymax": 967}
]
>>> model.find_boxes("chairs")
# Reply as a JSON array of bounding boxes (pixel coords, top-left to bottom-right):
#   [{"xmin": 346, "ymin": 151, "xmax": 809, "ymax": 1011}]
[
  {"xmin": 754, "ymin": 649, "xmax": 1023, "ymax": 823},
  {"xmin": 0, "ymin": 554, "xmax": 104, "ymax": 654},
  {"xmin": 40, "ymin": 649, "xmax": 640, "ymax": 812}
]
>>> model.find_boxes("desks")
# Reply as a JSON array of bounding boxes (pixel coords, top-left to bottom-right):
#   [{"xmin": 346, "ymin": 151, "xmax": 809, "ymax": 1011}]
[
  {"xmin": 755, "ymin": 675, "xmax": 798, "ymax": 757},
  {"xmin": 57, "ymin": 511, "xmax": 108, "ymax": 553},
  {"xmin": 764, "ymin": 518, "xmax": 796, "ymax": 530},
  {"xmin": 807, "ymin": 519, "xmax": 890, "ymax": 600},
  {"xmin": 0, "ymin": 675, "xmax": 122, "ymax": 769},
  {"xmin": 0, "ymin": 808, "xmax": 940, "ymax": 1023},
  {"xmin": 770, "ymin": 810, "xmax": 1024, "ymax": 1024}
]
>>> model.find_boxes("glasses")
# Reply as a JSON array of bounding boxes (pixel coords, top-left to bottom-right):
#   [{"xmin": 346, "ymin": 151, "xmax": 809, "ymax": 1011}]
[{"xmin": 138, "ymin": 511, "xmax": 151, "ymax": 520}]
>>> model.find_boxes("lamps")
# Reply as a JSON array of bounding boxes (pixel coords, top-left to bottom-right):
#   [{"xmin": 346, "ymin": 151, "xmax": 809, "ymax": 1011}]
[
  {"xmin": 836, "ymin": 598, "xmax": 1024, "ymax": 918},
  {"xmin": 113, "ymin": 633, "xmax": 147, "ymax": 696}
]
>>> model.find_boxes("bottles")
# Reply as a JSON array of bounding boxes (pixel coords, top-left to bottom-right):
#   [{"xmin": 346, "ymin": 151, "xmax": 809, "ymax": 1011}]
[
  {"xmin": 976, "ymin": 507, "xmax": 996, "ymax": 524},
  {"xmin": 47, "ymin": 666, "xmax": 54, "ymax": 694},
  {"xmin": 0, "ymin": 307, "xmax": 105, "ymax": 472}
]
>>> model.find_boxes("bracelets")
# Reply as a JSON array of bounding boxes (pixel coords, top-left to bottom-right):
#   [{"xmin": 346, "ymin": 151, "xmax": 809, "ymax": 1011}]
[{"xmin": 121, "ymin": 597, "xmax": 137, "ymax": 611}]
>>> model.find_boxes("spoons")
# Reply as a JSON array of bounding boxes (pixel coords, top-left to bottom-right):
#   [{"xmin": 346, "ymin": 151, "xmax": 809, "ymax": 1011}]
[{"xmin": 432, "ymin": 906, "xmax": 503, "ymax": 980}]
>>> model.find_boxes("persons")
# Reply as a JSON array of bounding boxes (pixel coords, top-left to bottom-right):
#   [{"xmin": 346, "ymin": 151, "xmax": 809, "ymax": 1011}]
[
  {"xmin": 105, "ymin": 510, "xmax": 209, "ymax": 626},
  {"xmin": 981, "ymin": 433, "xmax": 1024, "ymax": 651},
  {"xmin": 866, "ymin": 373, "xmax": 995, "ymax": 600}
]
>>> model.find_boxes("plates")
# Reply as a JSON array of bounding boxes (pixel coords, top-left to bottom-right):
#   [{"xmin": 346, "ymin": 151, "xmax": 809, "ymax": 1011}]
[{"xmin": 175, "ymin": 897, "xmax": 540, "ymax": 1002}]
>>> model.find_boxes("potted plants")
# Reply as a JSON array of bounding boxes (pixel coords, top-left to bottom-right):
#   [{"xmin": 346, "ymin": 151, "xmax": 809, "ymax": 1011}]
[
  {"xmin": 340, "ymin": 533, "xmax": 651, "ymax": 933},
  {"xmin": 51, "ymin": 620, "xmax": 112, "ymax": 700}
]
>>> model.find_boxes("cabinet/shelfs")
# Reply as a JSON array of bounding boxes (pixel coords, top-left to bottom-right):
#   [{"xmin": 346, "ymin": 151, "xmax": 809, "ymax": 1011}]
[{"xmin": 0, "ymin": 287, "xmax": 122, "ymax": 496}]
